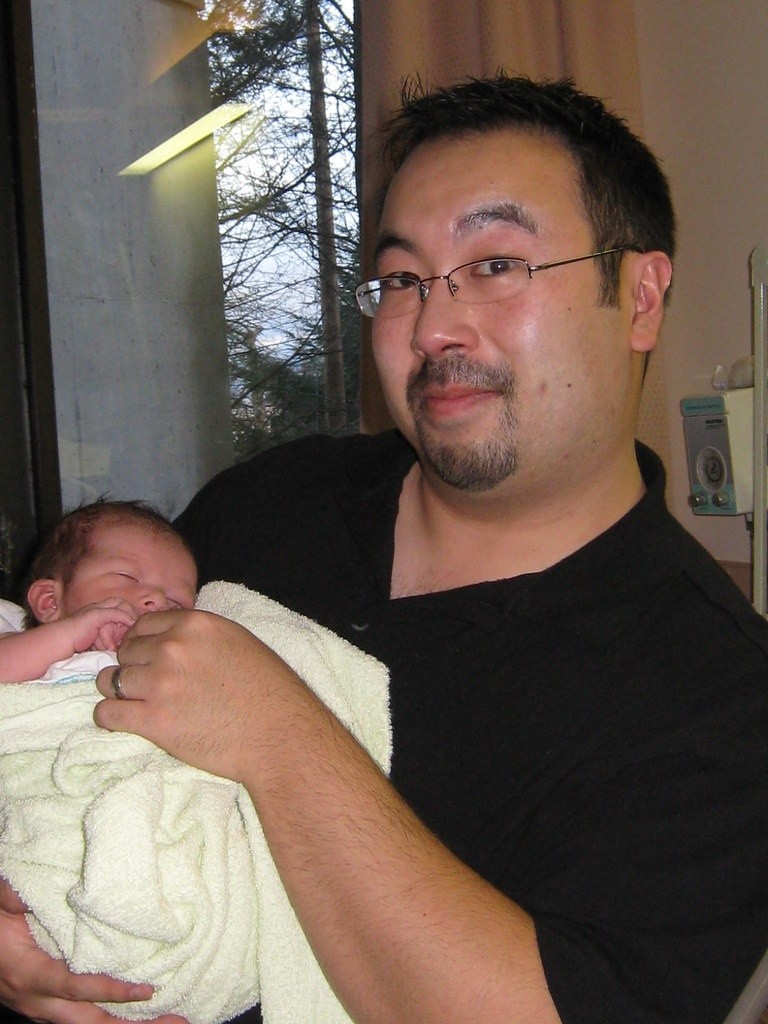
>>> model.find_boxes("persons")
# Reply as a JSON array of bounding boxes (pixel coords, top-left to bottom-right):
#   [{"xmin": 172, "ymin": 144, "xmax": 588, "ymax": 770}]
[
  {"xmin": 0, "ymin": 501, "xmax": 243, "ymax": 1018},
  {"xmin": 171, "ymin": 74, "xmax": 768, "ymax": 1024}
]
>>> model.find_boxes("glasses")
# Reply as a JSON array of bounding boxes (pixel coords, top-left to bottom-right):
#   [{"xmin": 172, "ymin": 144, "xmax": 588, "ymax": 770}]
[{"xmin": 351, "ymin": 244, "xmax": 645, "ymax": 320}]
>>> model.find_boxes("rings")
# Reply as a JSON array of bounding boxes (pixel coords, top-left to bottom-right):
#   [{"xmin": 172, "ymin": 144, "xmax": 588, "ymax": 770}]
[{"xmin": 111, "ymin": 664, "xmax": 132, "ymax": 700}]
